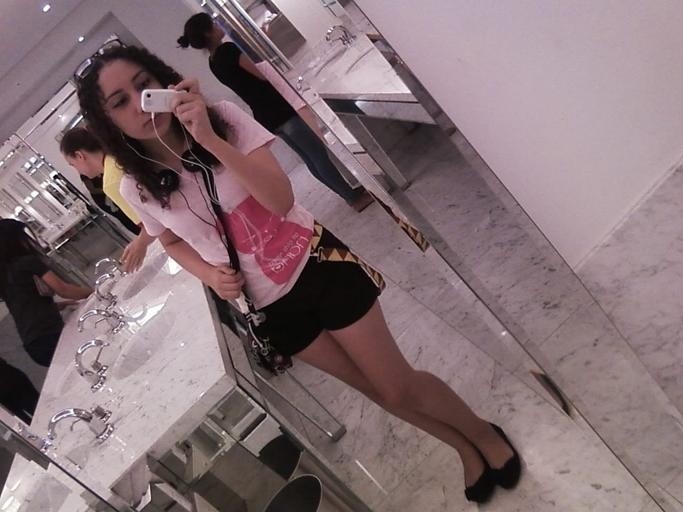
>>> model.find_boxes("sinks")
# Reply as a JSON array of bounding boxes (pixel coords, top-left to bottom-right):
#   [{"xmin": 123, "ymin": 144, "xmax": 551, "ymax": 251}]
[
  {"xmin": 82, "ymin": 279, "xmax": 117, "ymax": 312},
  {"xmin": 74, "ymin": 338, "xmax": 111, "ymax": 394},
  {"xmin": 122, "ymin": 250, "xmax": 169, "ymax": 301},
  {"xmin": 111, "ymin": 306, "xmax": 178, "ymax": 379},
  {"xmin": 57, "ymin": 333, "xmax": 113, "ymax": 396},
  {"xmin": 313, "ymin": 45, "xmax": 347, "ymax": 79},
  {"xmin": 345, "ymin": 45, "xmax": 374, "ymax": 75},
  {"xmin": 14, "ymin": 446, "xmax": 90, "ymax": 510}
]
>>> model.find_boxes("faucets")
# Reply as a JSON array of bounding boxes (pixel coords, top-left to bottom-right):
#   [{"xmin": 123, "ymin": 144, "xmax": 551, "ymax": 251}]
[
  {"xmin": 95, "ymin": 258, "xmax": 126, "ymax": 277},
  {"xmin": 77, "ymin": 308, "xmax": 125, "ymax": 335},
  {"xmin": 44, "ymin": 408, "xmax": 114, "ymax": 446},
  {"xmin": 325, "ymin": 25, "xmax": 357, "ymax": 47},
  {"xmin": 94, "ymin": 272, "xmax": 118, "ymax": 307}
]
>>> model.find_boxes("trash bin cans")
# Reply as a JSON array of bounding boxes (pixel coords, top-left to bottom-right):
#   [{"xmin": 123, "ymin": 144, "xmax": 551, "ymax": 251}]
[
  {"xmin": 257, "ymin": 435, "xmax": 361, "ymax": 511},
  {"xmin": 263, "ymin": 474, "xmax": 358, "ymax": 512}
]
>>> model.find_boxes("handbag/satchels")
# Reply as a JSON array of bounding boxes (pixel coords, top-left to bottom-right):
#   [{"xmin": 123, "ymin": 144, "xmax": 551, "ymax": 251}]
[
  {"xmin": 240, "ymin": 313, "xmax": 293, "ymax": 376},
  {"xmin": 31, "ymin": 272, "xmax": 55, "ymax": 297}
]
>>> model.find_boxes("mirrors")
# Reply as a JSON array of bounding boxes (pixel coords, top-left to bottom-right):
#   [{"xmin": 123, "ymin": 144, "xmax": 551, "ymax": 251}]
[
  {"xmin": 0, "ymin": 1, "xmax": 666, "ymax": 512},
  {"xmin": 0, "ymin": 135, "xmax": 135, "ymax": 492}
]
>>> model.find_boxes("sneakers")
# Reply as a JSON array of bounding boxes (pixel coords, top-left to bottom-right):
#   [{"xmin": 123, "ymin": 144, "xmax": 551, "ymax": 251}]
[
  {"xmin": 346, "ymin": 184, "xmax": 374, "ymax": 213},
  {"xmin": 463, "ymin": 461, "xmax": 494, "ymax": 504},
  {"xmin": 486, "ymin": 418, "xmax": 521, "ymax": 489}
]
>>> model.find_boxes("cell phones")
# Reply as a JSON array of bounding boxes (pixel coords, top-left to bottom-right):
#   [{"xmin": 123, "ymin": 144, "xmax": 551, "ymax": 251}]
[{"xmin": 140, "ymin": 89, "xmax": 188, "ymax": 112}]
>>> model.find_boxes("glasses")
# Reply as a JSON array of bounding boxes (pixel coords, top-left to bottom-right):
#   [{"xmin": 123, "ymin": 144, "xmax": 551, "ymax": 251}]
[{"xmin": 72, "ymin": 34, "xmax": 123, "ymax": 82}]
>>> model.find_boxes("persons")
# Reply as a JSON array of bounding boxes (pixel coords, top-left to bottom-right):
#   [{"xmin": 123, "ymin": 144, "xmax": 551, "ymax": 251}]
[
  {"xmin": 0, "ymin": 355, "xmax": 41, "ymax": 427},
  {"xmin": 0, "ymin": 216, "xmax": 95, "ymax": 370},
  {"xmin": 59, "ymin": 125, "xmax": 295, "ymax": 374},
  {"xmin": 176, "ymin": 12, "xmax": 375, "ymax": 213},
  {"xmin": 74, "ymin": 40, "xmax": 524, "ymax": 505}
]
